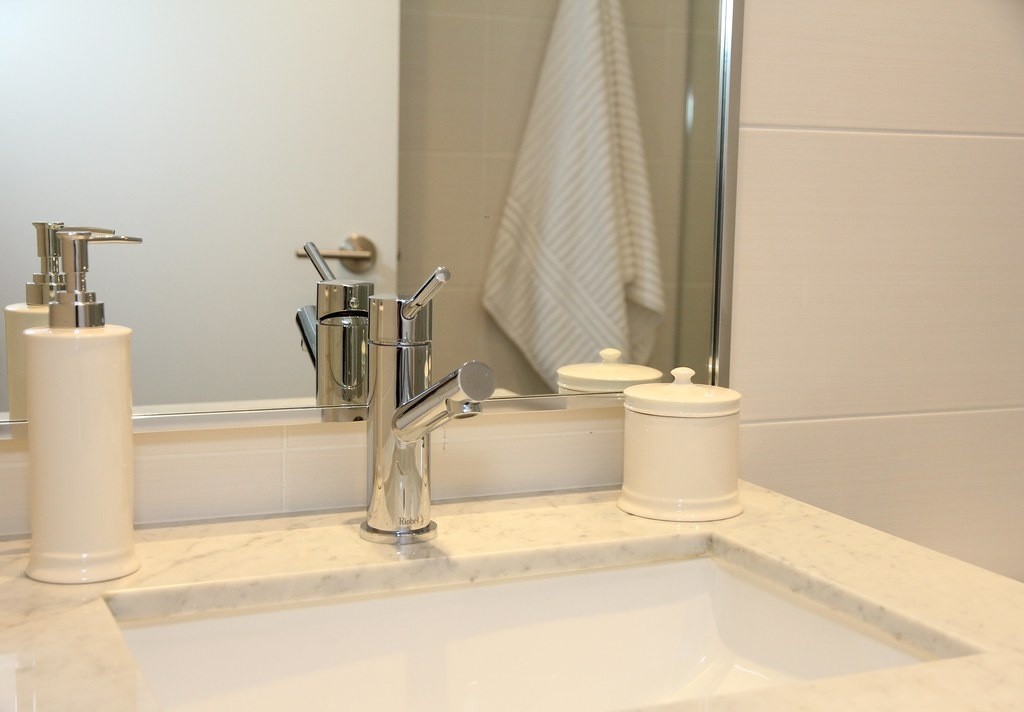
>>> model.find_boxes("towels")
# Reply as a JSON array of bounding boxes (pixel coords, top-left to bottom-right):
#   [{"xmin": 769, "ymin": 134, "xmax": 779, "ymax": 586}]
[{"xmin": 476, "ymin": 0, "xmax": 667, "ymax": 392}]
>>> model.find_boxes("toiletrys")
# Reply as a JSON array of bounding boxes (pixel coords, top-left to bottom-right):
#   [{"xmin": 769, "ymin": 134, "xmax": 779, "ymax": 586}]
[
  {"xmin": 6, "ymin": 218, "xmax": 115, "ymax": 422},
  {"xmin": 23, "ymin": 227, "xmax": 145, "ymax": 585}
]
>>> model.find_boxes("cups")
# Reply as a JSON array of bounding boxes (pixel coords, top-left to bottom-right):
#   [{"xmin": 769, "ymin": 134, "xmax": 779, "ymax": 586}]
[
  {"xmin": 618, "ymin": 365, "xmax": 743, "ymax": 523},
  {"xmin": 557, "ymin": 347, "xmax": 662, "ymax": 395}
]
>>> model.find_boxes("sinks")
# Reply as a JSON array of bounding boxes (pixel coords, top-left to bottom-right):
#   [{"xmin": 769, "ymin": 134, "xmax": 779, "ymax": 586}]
[{"xmin": 102, "ymin": 533, "xmax": 989, "ymax": 709}]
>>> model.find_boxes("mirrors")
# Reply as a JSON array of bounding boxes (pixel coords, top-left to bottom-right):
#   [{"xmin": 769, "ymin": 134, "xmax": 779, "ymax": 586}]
[{"xmin": 0, "ymin": 1, "xmax": 742, "ymax": 432}]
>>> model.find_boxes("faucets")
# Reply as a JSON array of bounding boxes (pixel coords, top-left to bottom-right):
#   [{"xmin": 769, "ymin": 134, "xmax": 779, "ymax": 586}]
[
  {"xmin": 293, "ymin": 239, "xmax": 377, "ymax": 422},
  {"xmin": 357, "ymin": 269, "xmax": 497, "ymax": 547}
]
